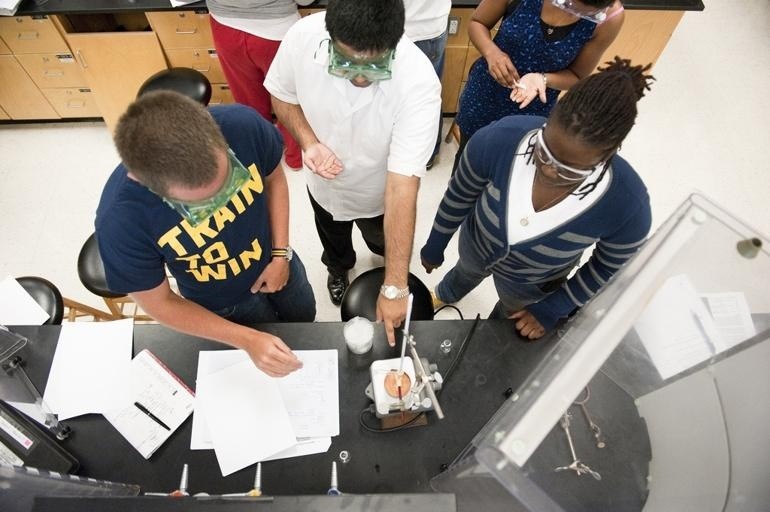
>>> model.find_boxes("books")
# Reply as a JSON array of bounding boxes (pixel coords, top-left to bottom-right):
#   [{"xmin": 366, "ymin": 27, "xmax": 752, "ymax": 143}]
[
  {"xmin": 96, "ymin": 349, "xmax": 196, "ymax": 460},
  {"xmin": 0, "ymin": 1, "xmax": 21, "ymax": 16}
]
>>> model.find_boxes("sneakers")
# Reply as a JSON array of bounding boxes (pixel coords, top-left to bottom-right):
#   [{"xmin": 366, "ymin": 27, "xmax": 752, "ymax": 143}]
[{"xmin": 428, "ymin": 286, "xmax": 443, "ymax": 309}]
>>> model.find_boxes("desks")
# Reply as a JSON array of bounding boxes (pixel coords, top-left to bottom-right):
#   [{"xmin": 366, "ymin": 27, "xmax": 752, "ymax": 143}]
[{"xmin": 1, "ymin": 317, "xmax": 652, "ymax": 512}]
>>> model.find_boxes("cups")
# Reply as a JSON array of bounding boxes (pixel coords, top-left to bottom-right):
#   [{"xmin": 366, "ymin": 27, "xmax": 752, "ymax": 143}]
[{"xmin": 342, "ymin": 315, "xmax": 375, "ymax": 355}]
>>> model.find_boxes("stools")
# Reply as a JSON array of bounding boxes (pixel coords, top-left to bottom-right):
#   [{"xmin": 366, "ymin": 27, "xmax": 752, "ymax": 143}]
[
  {"xmin": 340, "ymin": 266, "xmax": 434, "ymax": 321},
  {"xmin": 77, "ymin": 230, "xmax": 158, "ymax": 322},
  {"xmin": 12, "ymin": 277, "xmax": 118, "ymax": 325}
]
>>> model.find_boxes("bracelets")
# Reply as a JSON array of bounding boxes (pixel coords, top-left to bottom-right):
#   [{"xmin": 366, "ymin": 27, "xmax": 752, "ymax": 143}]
[{"xmin": 541, "ymin": 71, "xmax": 548, "ymax": 86}]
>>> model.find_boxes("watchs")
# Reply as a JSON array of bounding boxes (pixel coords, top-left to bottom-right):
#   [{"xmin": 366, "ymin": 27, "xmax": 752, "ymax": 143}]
[
  {"xmin": 271, "ymin": 245, "xmax": 293, "ymax": 261},
  {"xmin": 380, "ymin": 285, "xmax": 410, "ymax": 300}
]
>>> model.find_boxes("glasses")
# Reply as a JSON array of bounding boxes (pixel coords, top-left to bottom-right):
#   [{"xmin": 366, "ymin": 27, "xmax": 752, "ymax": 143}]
[
  {"xmin": 141, "ymin": 146, "xmax": 251, "ymax": 229},
  {"xmin": 534, "ymin": 121, "xmax": 596, "ymax": 185},
  {"xmin": 327, "ymin": 46, "xmax": 394, "ymax": 83}
]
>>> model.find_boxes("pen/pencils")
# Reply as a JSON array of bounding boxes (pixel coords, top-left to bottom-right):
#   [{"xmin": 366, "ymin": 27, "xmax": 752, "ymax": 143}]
[{"xmin": 134, "ymin": 402, "xmax": 172, "ymax": 431}]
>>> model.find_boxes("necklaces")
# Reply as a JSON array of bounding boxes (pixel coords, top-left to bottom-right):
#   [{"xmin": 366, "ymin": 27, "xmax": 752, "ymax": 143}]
[
  {"xmin": 547, "ymin": 7, "xmax": 570, "ymax": 35},
  {"xmin": 533, "ymin": 170, "xmax": 580, "ymax": 212}
]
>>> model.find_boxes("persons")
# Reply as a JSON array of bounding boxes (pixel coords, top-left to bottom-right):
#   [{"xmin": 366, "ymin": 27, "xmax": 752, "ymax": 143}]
[
  {"xmin": 263, "ymin": 0, "xmax": 442, "ymax": 349},
  {"xmin": 205, "ymin": 0, "xmax": 315, "ymax": 171},
  {"xmin": 451, "ymin": 0, "xmax": 624, "ymax": 176},
  {"xmin": 420, "ymin": 56, "xmax": 656, "ymax": 341},
  {"xmin": 403, "ymin": 0, "xmax": 453, "ymax": 171},
  {"xmin": 95, "ymin": 90, "xmax": 315, "ymax": 378}
]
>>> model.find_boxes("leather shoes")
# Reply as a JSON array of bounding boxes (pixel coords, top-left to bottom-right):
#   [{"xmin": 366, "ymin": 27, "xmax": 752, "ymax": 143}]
[{"xmin": 326, "ymin": 272, "xmax": 350, "ymax": 307}]
